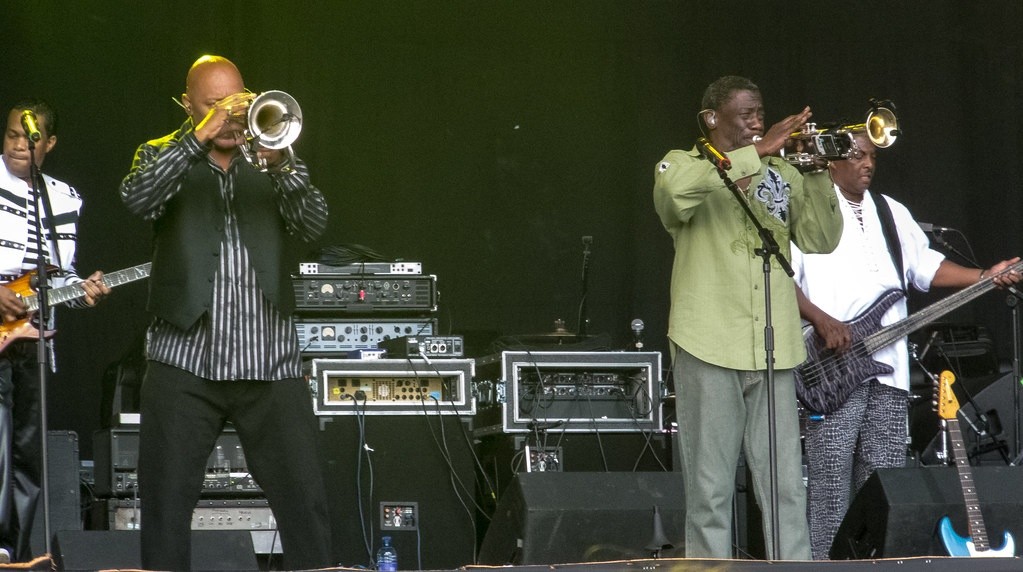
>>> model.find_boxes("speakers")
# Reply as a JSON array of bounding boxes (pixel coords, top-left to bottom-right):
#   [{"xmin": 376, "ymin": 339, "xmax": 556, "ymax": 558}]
[
  {"xmin": 319, "ymin": 413, "xmax": 476, "ymax": 572},
  {"xmin": 479, "ymin": 472, "xmax": 687, "ymax": 566},
  {"xmin": 921, "ymin": 369, "xmax": 1023, "ymax": 467},
  {"xmin": 37, "ymin": 530, "xmax": 260, "ymax": 572},
  {"xmin": 829, "ymin": 467, "xmax": 1023, "ymax": 561},
  {"xmin": 20, "ymin": 429, "xmax": 81, "ymax": 572}
]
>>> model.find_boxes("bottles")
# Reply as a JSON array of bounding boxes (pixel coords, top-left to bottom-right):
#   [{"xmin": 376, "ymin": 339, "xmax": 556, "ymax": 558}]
[{"xmin": 376, "ymin": 536, "xmax": 398, "ymax": 572}]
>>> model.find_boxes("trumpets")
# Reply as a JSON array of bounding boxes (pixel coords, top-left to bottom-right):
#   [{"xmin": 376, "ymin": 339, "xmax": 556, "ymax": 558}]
[
  {"xmin": 231, "ymin": 89, "xmax": 305, "ymax": 177},
  {"xmin": 751, "ymin": 104, "xmax": 901, "ymax": 168}
]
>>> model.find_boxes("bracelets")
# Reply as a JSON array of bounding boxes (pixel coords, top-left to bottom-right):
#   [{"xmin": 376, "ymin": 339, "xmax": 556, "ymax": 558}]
[{"xmin": 979, "ymin": 269, "xmax": 986, "ymax": 279}]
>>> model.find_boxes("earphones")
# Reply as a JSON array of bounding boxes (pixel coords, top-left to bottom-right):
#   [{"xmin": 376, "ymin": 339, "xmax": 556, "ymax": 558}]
[{"xmin": 707, "ymin": 116, "xmax": 715, "ymax": 127}]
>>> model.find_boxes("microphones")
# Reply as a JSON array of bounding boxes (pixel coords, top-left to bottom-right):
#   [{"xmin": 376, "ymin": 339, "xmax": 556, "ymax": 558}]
[
  {"xmin": 631, "ymin": 319, "xmax": 644, "ymax": 352},
  {"xmin": 918, "ymin": 332, "xmax": 938, "ymax": 362},
  {"xmin": 696, "ymin": 137, "xmax": 732, "ymax": 171},
  {"xmin": 918, "ymin": 222, "xmax": 955, "ymax": 232},
  {"xmin": 281, "ymin": 114, "xmax": 290, "ymax": 121},
  {"xmin": 20, "ymin": 110, "xmax": 41, "ymax": 142}
]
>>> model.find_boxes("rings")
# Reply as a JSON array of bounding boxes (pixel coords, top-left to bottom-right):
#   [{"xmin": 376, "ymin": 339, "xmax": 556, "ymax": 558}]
[
  {"xmin": 16, "ymin": 292, "xmax": 21, "ymax": 298},
  {"xmin": 226, "ymin": 106, "xmax": 233, "ymax": 116}
]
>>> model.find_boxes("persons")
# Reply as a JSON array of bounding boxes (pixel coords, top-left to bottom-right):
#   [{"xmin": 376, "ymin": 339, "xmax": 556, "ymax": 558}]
[
  {"xmin": 654, "ymin": 76, "xmax": 844, "ymax": 563},
  {"xmin": 0, "ymin": 100, "xmax": 113, "ymax": 564},
  {"xmin": 789, "ymin": 132, "xmax": 1023, "ymax": 560},
  {"xmin": 120, "ymin": 56, "xmax": 335, "ymax": 571}
]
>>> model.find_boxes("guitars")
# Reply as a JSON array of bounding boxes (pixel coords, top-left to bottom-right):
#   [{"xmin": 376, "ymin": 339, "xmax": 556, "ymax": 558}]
[
  {"xmin": 0, "ymin": 261, "xmax": 153, "ymax": 356},
  {"xmin": 794, "ymin": 258, "xmax": 1023, "ymax": 416},
  {"xmin": 929, "ymin": 367, "xmax": 1017, "ymax": 559}
]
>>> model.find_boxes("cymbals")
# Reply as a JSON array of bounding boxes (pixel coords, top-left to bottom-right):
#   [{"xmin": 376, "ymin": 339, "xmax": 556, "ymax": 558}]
[{"xmin": 500, "ymin": 330, "xmax": 597, "ymax": 342}]
